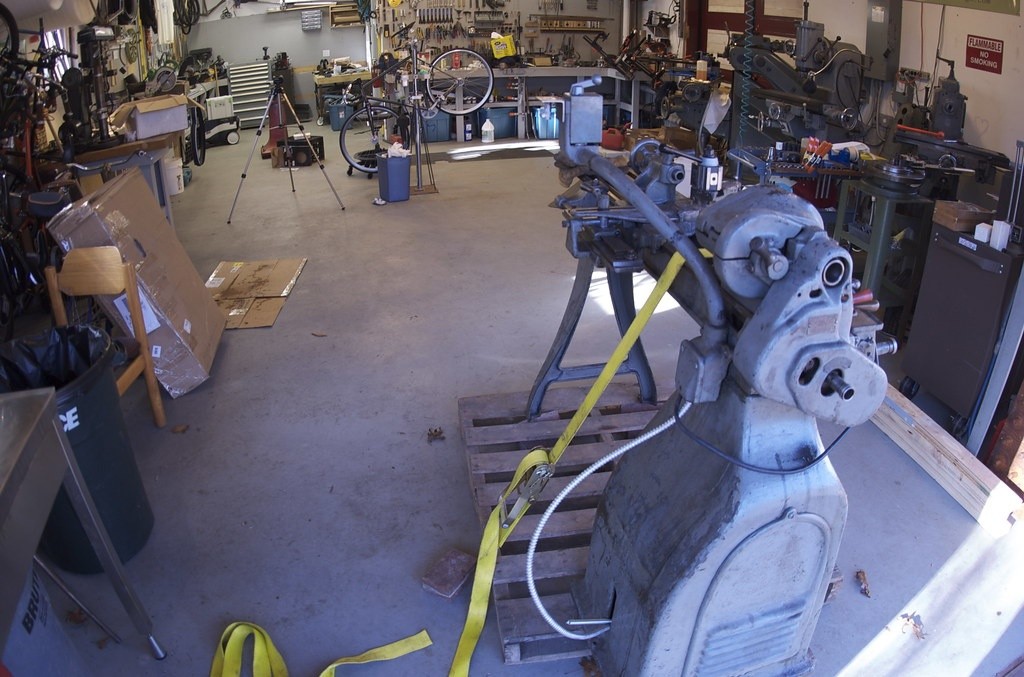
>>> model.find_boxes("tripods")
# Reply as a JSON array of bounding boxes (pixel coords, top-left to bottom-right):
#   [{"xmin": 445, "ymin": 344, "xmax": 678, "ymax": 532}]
[{"xmin": 227, "ymin": 74, "xmax": 346, "ymax": 224}]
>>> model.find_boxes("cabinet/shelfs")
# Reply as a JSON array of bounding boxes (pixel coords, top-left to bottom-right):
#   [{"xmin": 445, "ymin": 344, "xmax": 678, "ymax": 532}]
[
  {"xmin": 831, "ymin": 178, "xmax": 935, "ymax": 341},
  {"xmin": 384, "ymin": 65, "xmax": 673, "ymax": 130}
]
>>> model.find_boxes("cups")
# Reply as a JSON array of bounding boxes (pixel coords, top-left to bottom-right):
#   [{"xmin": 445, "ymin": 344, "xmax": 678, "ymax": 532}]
[{"xmin": 334, "ymin": 66, "xmax": 341, "ymax": 75}]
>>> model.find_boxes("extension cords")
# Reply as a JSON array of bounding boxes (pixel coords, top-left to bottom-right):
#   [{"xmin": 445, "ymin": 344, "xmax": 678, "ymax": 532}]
[{"xmin": 900, "ymin": 67, "xmax": 930, "ymax": 79}]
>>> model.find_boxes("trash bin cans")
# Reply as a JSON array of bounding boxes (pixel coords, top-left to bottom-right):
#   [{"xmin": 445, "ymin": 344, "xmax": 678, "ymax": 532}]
[
  {"xmin": 376, "ymin": 152, "xmax": 413, "ymax": 203},
  {"xmin": 0, "ymin": 325, "xmax": 154, "ymax": 577},
  {"xmin": 328, "ymin": 103, "xmax": 353, "ymax": 131}
]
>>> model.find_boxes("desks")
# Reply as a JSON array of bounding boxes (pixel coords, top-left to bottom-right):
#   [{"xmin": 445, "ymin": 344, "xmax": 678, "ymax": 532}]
[
  {"xmin": 0, "ymin": 388, "xmax": 166, "ymax": 667},
  {"xmin": 314, "ymin": 70, "xmax": 371, "ymax": 121}
]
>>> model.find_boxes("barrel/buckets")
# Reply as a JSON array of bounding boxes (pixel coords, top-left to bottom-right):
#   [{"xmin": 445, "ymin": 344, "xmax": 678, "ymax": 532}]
[{"xmin": 165, "ymin": 157, "xmax": 184, "ymax": 195}]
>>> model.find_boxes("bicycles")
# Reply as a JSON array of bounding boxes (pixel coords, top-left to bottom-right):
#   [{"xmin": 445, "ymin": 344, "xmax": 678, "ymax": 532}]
[
  {"xmin": 0, "ymin": 1, "xmax": 155, "ymax": 374},
  {"xmin": 338, "ymin": 36, "xmax": 495, "ymax": 175}
]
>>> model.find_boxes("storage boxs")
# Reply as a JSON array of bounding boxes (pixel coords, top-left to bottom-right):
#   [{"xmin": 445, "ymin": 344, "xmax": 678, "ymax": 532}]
[
  {"xmin": 417, "ymin": 111, "xmax": 450, "ymax": 141},
  {"xmin": 931, "ymin": 199, "xmax": 996, "ymax": 234},
  {"xmin": 329, "ymin": 104, "xmax": 354, "ymax": 131},
  {"xmin": 473, "ymin": 107, "xmax": 518, "ymax": 139},
  {"xmin": 533, "ymin": 107, "xmax": 560, "ymax": 139},
  {"xmin": 525, "ymin": 54, "xmax": 552, "ymax": 66},
  {"xmin": 848, "ymin": 222, "xmax": 871, "ymax": 243},
  {"xmin": 376, "ymin": 150, "xmax": 411, "ymax": 203},
  {"xmin": 48, "ymin": 165, "xmax": 224, "ymax": 400},
  {"xmin": 109, "ymin": 91, "xmax": 191, "ymax": 142}
]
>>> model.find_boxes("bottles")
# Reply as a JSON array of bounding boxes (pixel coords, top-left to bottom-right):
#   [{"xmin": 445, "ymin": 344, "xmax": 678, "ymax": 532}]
[
  {"xmin": 697, "ymin": 60, "xmax": 707, "ymax": 80},
  {"xmin": 710, "ymin": 61, "xmax": 720, "ymax": 81},
  {"xmin": 481, "ymin": 119, "xmax": 494, "ymax": 143}
]
